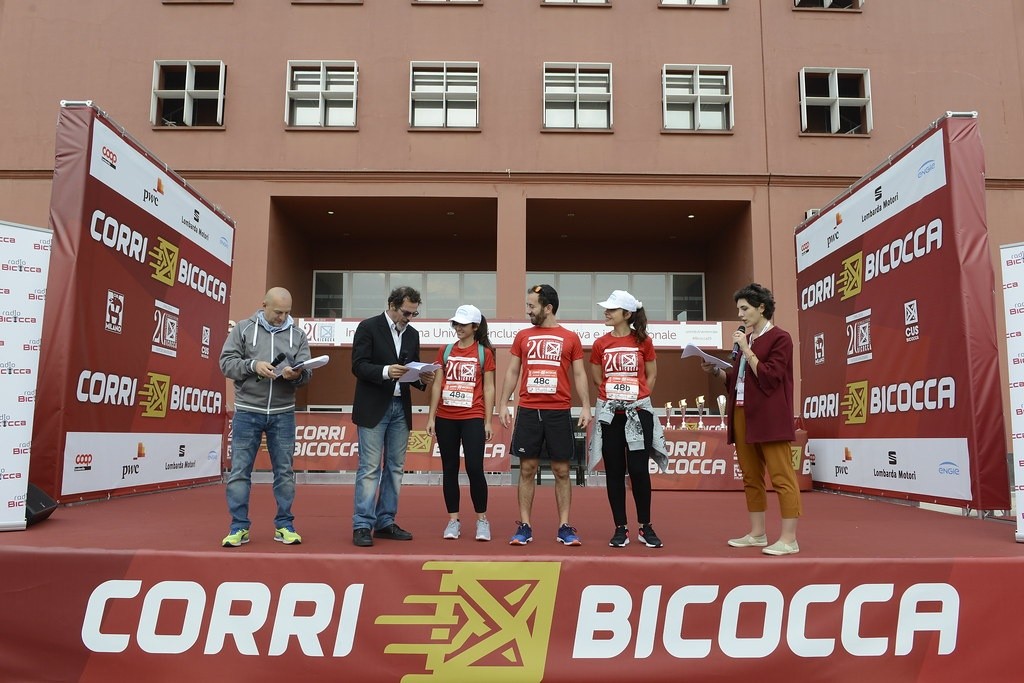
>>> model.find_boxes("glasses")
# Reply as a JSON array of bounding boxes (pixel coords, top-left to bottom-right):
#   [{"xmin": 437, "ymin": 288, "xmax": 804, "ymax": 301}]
[
  {"xmin": 450, "ymin": 320, "xmax": 469, "ymax": 326},
  {"xmin": 528, "ymin": 284, "xmax": 547, "ymax": 298},
  {"xmin": 397, "ymin": 306, "xmax": 419, "ymax": 317}
]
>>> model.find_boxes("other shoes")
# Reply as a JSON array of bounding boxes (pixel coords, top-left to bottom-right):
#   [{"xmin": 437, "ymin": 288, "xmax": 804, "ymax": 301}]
[
  {"xmin": 727, "ymin": 533, "xmax": 768, "ymax": 548},
  {"xmin": 761, "ymin": 539, "xmax": 800, "ymax": 555}
]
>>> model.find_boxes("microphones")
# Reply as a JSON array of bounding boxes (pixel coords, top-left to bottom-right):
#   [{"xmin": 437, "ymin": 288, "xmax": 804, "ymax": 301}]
[
  {"xmin": 731, "ymin": 326, "xmax": 745, "ymax": 360},
  {"xmin": 255, "ymin": 352, "xmax": 286, "ymax": 382},
  {"xmin": 392, "ymin": 350, "xmax": 409, "ymax": 382}
]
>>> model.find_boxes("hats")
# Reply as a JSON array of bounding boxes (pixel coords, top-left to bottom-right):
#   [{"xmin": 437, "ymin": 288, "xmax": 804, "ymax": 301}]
[
  {"xmin": 448, "ymin": 304, "xmax": 481, "ymax": 325},
  {"xmin": 596, "ymin": 290, "xmax": 637, "ymax": 312}
]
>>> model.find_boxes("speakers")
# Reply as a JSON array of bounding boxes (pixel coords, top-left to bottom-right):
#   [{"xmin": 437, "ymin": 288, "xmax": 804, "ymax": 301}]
[{"xmin": 26, "ymin": 482, "xmax": 58, "ymax": 525}]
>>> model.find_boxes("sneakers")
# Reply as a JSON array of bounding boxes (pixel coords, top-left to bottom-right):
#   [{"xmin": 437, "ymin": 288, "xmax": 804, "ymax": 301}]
[
  {"xmin": 221, "ymin": 527, "xmax": 250, "ymax": 547},
  {"xmin": 508, "ymin": 520, "xmax": 533, "ymax": 545},
  {"xmin": 353, "ymin": 528, "xmax": 373, "ymax": 547},
  {"xmin": 607, "ymin": 524, "xmax": 630, "ymax": 547},
  {"xmin": 372, "ymin": 523, "xmax": 412, "ymax": 540},
  {"xmin": 475, "ymin": 519, "xmax": 491, "ymax": 540},
  {"xmin": 556, "ymin": 523, "xmax": 582, "ymax": 546},
  {"xmin": 273, "ymin": 527, "xmax": 302, "ymax": 545},
  {"xmin": 637, "ymin": 524, "xmax": 664, "ymax": 548},
  {"xmin": 443, "ymin": 519, "xmax": 461, "ymax": 539}
]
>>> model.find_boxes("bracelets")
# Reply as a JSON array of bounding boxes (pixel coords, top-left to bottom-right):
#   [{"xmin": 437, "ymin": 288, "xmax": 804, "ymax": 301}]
[
  {"xmin": 713, "ymin": 368, "xmax": 720, "ymax": 376},
  {"xmin": 746, "ymin": 355, "xmax": 755, "ymax": 363}
]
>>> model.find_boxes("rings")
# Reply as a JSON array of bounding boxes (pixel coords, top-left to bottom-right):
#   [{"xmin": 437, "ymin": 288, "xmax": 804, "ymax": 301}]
[{"xmin": 738, "ymin": 335, "xmax": 740, "ymax": 337}]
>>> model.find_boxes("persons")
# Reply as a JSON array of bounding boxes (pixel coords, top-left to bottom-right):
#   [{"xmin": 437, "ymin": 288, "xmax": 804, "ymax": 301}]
[
  {"xmin": 219, "ymin": 287, "xmax": 312, "ymax": 546},
  {"xmin": 700, "ymin": 283, "xmax": 802, "ymax": 555},
  {"xmin": 588, "ymin": 289, "xmax": 669, "ymax": 548},
  {"xmin": 426, "ymin": 304, "xmax": 495, "ymax": 540},
  {"xmin": 498, "ymin": 285, "xmax": 591, "ymax": 545},
  {"xmin": 351, "ymin": 287, "xmax": 435, "ymax": 546}
]
